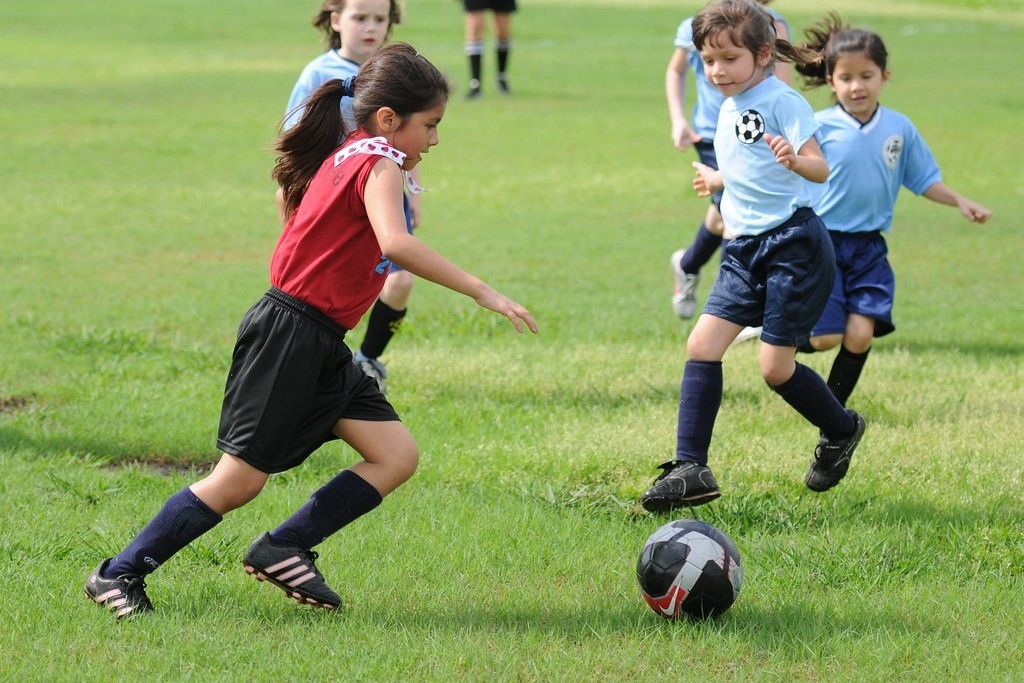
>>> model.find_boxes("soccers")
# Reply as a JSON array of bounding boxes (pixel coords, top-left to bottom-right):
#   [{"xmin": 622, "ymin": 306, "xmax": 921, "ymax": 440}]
[{"xmin": 635, "ymin": 519, "xmax": 744, "ymax": 623}]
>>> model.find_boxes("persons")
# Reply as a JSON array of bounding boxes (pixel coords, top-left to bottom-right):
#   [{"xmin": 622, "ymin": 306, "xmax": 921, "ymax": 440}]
[
  {"xmin": 464, "ymin": 0, "xmax": 517, "ymax": 97},
  {"xmin": 665, "ymin": 0, "xmax": 793, "ymax": 342},
  {"xmin": 775, "ymin": 8, "xmax": 992, "ymax": 437},
  {"xmin": 83, "ymin": 42, "xmax": 538, "ymax": 624},
  {"xmin": 643, "ymin": 0, "xmax": 866, "ymax": 515},
  {"xmin": 283, "ymin": 0, "xmax": 421, "ymax": 396}
]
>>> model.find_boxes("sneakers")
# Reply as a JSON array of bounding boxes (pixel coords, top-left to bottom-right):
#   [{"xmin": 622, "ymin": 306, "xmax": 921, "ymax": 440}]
[
  {"xmin": 732, "ymin": 326, "xmax": 763, "ymax": 345},
  {"xmin": 464, "ymin": 88, "xmax": 480, "ymax": 99},
  {"xmin": 499, "ymin": 80, "xmax": 508, "ymax": 93},
  {"xmin": 642, "ymin": 459, "xmax": 722, "ymax": 514},
  {"xmin": 243, "ymin": 531, "xmax": 342, "ymax": 610},
  {"xmin": 672, "ymin": 249, "xmax": 701, "ymax": 319},
  {"xmin": 353, "ymin": 350, "xmax": 388, "ymax": 394},
  {"xmin": 805, "ymin": 409, "xmax": 865, "ymax": 492},
  {"xmin": 83, "ymin": 558, "xmax": 155, "ymax": 624}
]
